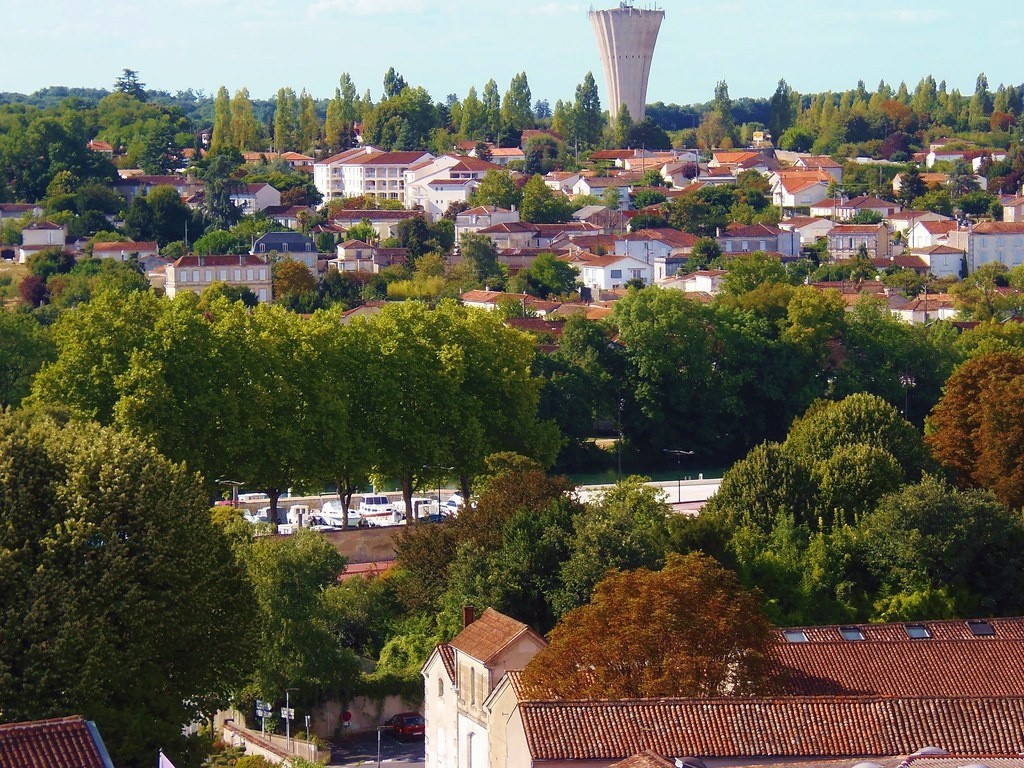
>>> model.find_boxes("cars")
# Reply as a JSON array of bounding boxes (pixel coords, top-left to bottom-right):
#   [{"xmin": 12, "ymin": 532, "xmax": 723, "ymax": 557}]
[
  {"xmin": 232, "ymin": 490, "xmax": 476, "ymax": 537},
  {"xmin": 384, "ymin": 712, "xmax": 425, "ymax": 742}
]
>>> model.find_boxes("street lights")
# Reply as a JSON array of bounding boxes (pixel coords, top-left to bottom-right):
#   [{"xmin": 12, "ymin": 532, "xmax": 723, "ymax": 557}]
[
  {"xmin": 213, "ymin": 479, "xmax": 246, "ymax": 508},
  {"xmin": 377, "ymin": 726, "xmax": 393, "ymax": 768},
  {"xmin": 422, "ymin": 463, "xmax": 460, "ymax": 524},
  {"xmin": 286, "ymin": 687, "xmax": 302, "ymax": 750},
  {"xmin": 663, "ymin": 449, "xmax": 696, "ymax": 503}
]
